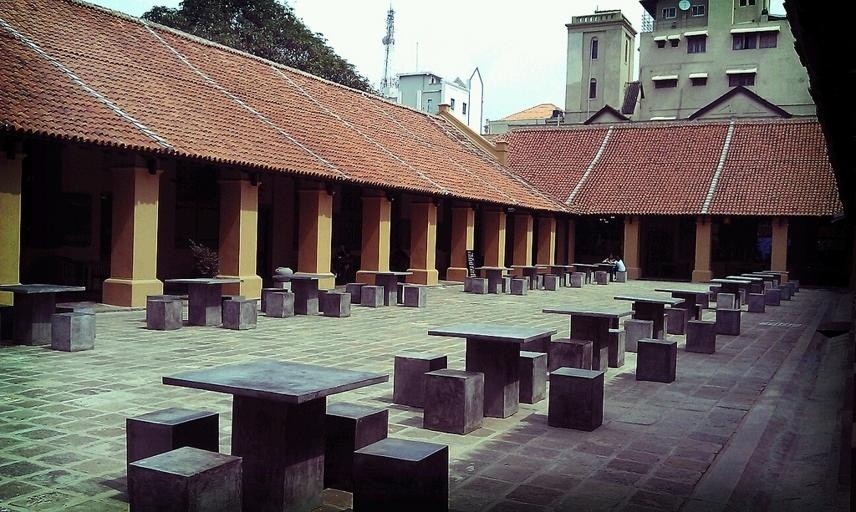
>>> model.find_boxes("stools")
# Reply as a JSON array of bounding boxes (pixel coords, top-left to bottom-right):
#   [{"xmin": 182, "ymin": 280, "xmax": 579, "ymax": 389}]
[
  {"xmin": 548, "ymin": 366, "xmax": 605, "ymax": 432},
  {"xmin": 608, "ymin": 270, "xmax": 799, "ymax": 383},
  {"xmin": 519, "ymin": 351, "xmax": 548, "ymax": 404},
  {"xmin": 393, "ymin": 352, "xmax": 447, "ymax": 408},
  {"xmin": 548, "ymin": 338, "xmax": 594, "ymax": 374},
  {"xmin": 126, "ymin": 407, "xmax": 219, "ymax": 485},
  {"xmin": 353, "ymin": 437, "xmax": 448, "ymax": 512},
  {"xmin": 129, "ymin": 445, "xmax": 243, "ymax": 512},
  {"xmin": 520, "ymin": 335, "xmax": 551, "ymax": 372},
  {"xmin": 424, "ymin": 368, "xmax": 485, "ymax": 434},
  {"xmin": 323, "ymin": 402, "xmax": 388, "ymax": 492}
]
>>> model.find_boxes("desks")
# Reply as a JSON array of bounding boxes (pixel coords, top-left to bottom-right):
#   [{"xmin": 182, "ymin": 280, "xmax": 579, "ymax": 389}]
[
  {"xmin": 464, "ymin": 263, "xmax": 627, "ymax": 295},
  {"xmin": 543, "ymin": 304, "xmax": 635, "ymax": 374},
  {"xmin": 163, "ymin": 358, "xmax": 389, "ymax": 512},
  {"xmin": 428, "ymin": 322, "xmax": 557, "ymax": 419}
]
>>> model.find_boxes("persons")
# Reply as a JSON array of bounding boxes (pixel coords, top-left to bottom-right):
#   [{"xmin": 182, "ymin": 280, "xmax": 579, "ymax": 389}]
[
  {"xmin": 611, "ymin": 255, "xmax": 625, "ymax": 279},
  {"xmin": 602, "ymin": 253, "xmax": 616, "ymax": 273},
  {"xmin": 334, "ymin": 243, "xmax": 351, "ymax": 278}
]
